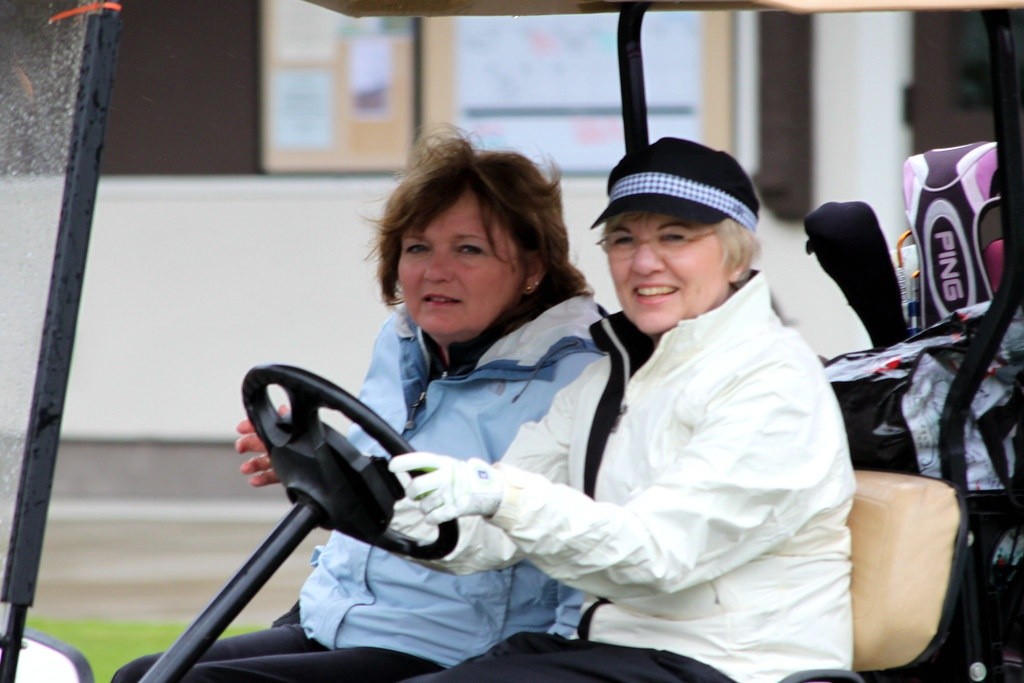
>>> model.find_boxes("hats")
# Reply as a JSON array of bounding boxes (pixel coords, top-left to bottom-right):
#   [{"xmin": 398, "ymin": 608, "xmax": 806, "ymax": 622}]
[{"xmin": 591, "ymin": 137, "xmax": 760, "ymax": 232}]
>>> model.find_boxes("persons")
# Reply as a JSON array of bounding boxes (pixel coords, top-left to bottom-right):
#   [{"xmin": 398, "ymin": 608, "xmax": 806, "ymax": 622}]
[
  {"xmin": 382, "ymin": 136, "xmax": 862, "ymax": 683},
  {"xmin": 102, "ymin": 136, "xmax": 611, "ymax": 682}
]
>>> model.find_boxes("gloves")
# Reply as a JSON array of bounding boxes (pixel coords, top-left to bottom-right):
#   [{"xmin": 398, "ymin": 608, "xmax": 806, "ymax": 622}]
[
  {"xmin": 388, "ymin": 451, "xmax": 501, "ymax": 526},
  {"xmin": 390, "ymin": 498, "xmax": 438, "ymax": 547}
]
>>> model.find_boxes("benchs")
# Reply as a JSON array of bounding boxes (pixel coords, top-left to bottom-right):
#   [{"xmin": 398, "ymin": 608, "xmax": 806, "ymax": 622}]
[{"xmin": 842, "ymin": 466, "xmax": 970, "ymax": 683}]
